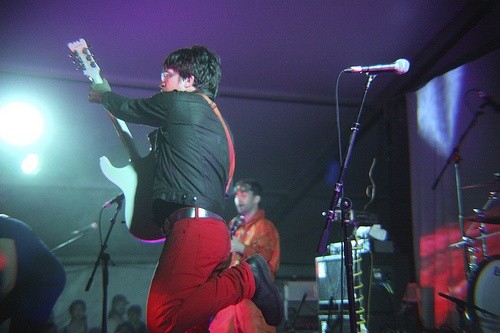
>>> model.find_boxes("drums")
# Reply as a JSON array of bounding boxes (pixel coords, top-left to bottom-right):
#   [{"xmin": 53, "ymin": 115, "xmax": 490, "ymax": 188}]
[{"xmin": 467, "ymin": 254, "xmax": 500, "ymax": 333}]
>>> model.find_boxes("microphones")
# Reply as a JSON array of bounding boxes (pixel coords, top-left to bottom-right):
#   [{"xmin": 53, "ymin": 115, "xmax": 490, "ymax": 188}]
[
  {"xmin": 477, "ymin": 90, "xmax": 500, "ymax": 114},
  {"xmin": 104, "ymin": 192, "xmax": 123, "ymax": 207},
  {"xmin": 346, "ymin": 58, "xmax": 410, "ymax": 74},
  {"xmin": 71, "ymin": 223, "xmax": 98, "ymax": 236}
]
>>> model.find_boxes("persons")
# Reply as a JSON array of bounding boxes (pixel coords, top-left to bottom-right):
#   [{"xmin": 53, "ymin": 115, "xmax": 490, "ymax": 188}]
[
  {"xmin": 47, "ymin": 294, "xmax": 148, "ymax": 333},
  {"xmin": 208, "ymin": 179, "xmax": 280, "ymax": 333},
  {"xmin": 89, "ymin": 45, "xmax": 283, "ymax": 333},
  {"xmin": 0, "ymin": 213, "xmax": 67, "ymax": 333}
]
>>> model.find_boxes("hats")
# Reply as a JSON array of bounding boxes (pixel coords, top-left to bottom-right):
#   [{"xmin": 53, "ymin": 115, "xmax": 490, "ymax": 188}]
[{"xmin": 112, "ymin": 294, "xmax": 129, "ymax": 305}]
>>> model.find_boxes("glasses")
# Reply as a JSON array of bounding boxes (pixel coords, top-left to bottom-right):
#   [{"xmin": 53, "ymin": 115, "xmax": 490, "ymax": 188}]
[
  {"xmin": 160, "ymin": 71, "xmax": 172, "ymax": 82},
  {"xmin": 233, "ymin": 184, "xmax": 255, "ymax": 193}
]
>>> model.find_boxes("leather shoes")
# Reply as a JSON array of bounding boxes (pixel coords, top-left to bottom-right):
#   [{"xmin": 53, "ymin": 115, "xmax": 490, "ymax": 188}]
[{"xmin": 242, "ymin": 253, "xmax": 284, "ymax": 328}]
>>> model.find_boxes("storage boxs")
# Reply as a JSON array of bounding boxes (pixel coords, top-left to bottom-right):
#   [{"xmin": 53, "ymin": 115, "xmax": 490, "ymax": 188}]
[{"xmin": 314, "ymin": 252, "xmax": 409, "ymax": 313}]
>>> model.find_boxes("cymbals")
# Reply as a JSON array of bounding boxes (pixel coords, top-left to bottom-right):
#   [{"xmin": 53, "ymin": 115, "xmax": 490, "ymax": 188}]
[
  {"xmin": 462, "ymin": 181, "xmax": 499, "ymax": 190},
  {"xmin": 464, "ymin": 215, "xmax": 500, "ymax": 224}
]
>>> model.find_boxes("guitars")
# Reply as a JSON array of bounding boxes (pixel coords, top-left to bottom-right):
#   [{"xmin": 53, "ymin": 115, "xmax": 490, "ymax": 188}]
[
  {"xmin": 67, "ymin": 38, "xmax": 168, "ymax": 243},
  {"xmin": 229, "ymin": 214, "xmax": 245, "ymax": 236}
]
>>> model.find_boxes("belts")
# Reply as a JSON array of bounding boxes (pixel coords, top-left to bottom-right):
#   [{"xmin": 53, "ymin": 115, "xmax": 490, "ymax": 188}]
[{"xmin": 159, "ymin": 206, "xmax": 225, "ymax": 237}]
